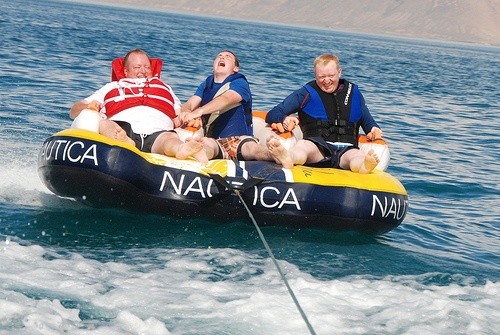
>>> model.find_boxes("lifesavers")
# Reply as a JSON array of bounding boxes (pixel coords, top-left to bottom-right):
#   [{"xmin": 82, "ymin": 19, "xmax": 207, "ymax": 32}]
[
  {"xmin": 200, "ymin": 73, "xmax": 254, "ymax": 140},
  {"xmin": 103, "ymin": 58, "xmax": 177, "ymax": 120},
  {"xmin": 298, "ymin": 79, "xmax": 361, "ymax": 148}
]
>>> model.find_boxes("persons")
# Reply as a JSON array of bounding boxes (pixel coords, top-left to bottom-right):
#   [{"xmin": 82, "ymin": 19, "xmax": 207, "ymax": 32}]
[
  {"xmin": 69, "ymin": 49, "xmax": 205, "ymax": 160},
  {"xmin": 180, "ymin": 50, "xmax": 281, "ymax": 164},
  {"xmin": 265, "ymin": 54, "xmax": 383, "ymax": 174}
]
